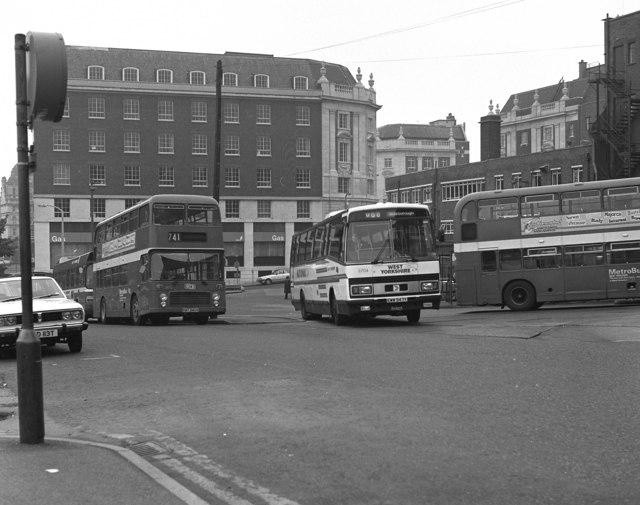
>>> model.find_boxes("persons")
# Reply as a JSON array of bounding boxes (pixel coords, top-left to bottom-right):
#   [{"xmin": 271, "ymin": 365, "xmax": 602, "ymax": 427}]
[{"xmin": 284, "ymin": 276, "xmax": 291, "ymax": 300}]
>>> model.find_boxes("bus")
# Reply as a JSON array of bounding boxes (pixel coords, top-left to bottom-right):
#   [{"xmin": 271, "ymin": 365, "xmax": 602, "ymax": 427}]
[
  {"xmin": 451, "ymin": 177, "xmax": 640, "ymax": 312},
  {"xmin": 52, "ymin": 250, "xmax": 94, "ymax": 320},
  {"xmin": 92, "ymin": 194, "xmax": 227, "ymax": 328},
  {"xmin": 289, "ymin": 201, "xmax": 441, "ymax": 326}
]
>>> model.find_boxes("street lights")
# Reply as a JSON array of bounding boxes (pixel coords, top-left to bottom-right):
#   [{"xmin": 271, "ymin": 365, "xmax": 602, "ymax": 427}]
[{"xmin": 37, "ymin": 203, "xmax": 65, "ymax": 256}]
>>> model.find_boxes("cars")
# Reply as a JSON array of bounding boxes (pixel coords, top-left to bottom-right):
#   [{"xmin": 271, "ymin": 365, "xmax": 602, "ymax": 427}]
[
  {"xmin": 0, "ymin": 276, "xmax": 89, "ymax": 354},
  {"xmin": 256, "ymin": 268, "xmax": 290, "ymax": 285}
]
